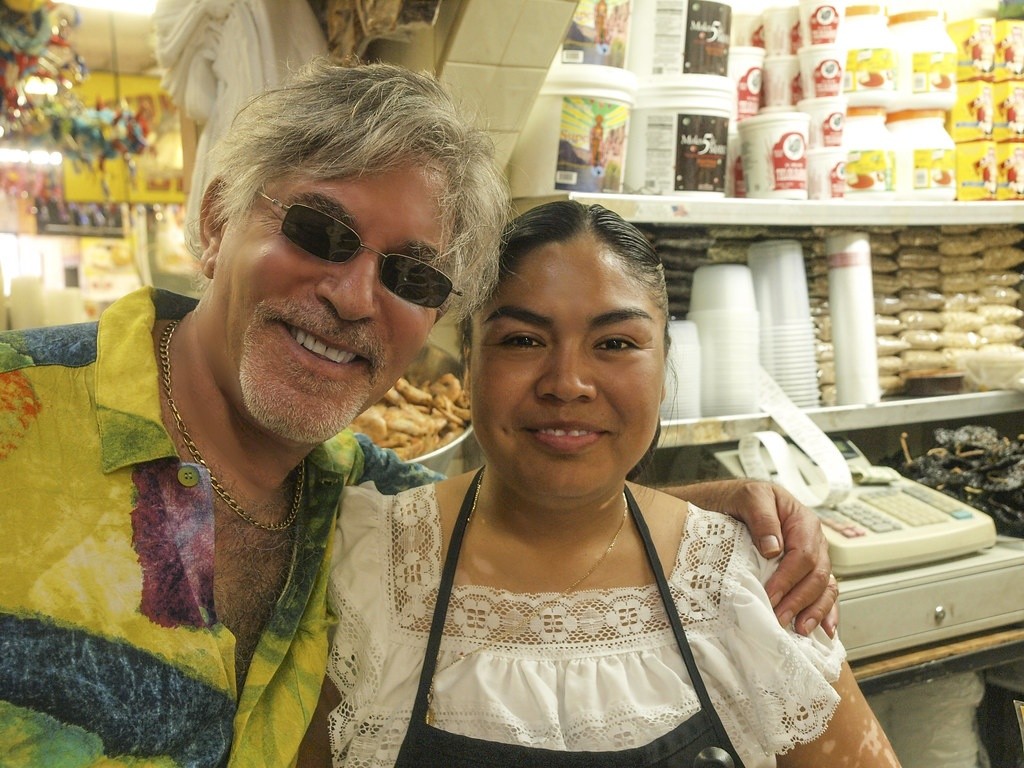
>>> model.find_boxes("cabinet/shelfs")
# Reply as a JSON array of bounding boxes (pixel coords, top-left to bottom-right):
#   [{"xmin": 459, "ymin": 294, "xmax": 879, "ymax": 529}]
[{"xmin": 1, "ymin": 0, "xmax": 1024, "ymax": 768}]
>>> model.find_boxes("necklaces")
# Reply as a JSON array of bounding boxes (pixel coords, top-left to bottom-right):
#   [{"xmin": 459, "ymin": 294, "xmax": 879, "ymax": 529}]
[
  {"xmin": 161, "ymin": 318, "xmax": 306, "ymax": 529},
  {"xmin": 426, "ymin": 468, "xmax": 628, "ymax": 726}
]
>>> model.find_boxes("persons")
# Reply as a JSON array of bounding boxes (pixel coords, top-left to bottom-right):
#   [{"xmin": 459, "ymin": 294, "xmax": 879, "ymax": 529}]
[
  {"xmin": 1, "ymin": 66, "xmax": 840, "ymax": 768},
  {"xmin": 298, "ymin": 200, "xmax": 904, "ymax": 768}
]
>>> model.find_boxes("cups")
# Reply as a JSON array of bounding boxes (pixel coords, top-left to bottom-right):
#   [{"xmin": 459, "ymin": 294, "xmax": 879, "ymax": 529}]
[
  {"xmin": 729, "ymin": 0, "xmax": 848, "ymax": 201},
  {"xmin": 657, "ymin": 239, "xmax": 822, "ymax": 422}
]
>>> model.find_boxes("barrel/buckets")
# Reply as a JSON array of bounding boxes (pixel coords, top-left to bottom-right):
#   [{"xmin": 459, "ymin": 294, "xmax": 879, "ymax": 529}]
[{"xmin": 506, "ymin": 1, "xmax": 734, "ymax": 200}]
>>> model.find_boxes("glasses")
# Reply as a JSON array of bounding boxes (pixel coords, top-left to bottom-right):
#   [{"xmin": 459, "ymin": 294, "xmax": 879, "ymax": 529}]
[{"xmin": 256, "ymin": 190, "xmax": 463, "ymax": 308}]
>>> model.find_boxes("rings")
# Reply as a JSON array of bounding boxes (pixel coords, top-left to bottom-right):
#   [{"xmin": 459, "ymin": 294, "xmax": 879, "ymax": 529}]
[{"xmin": 830, "ymin": 575, "xmax": 834, "ymax": 577}]
